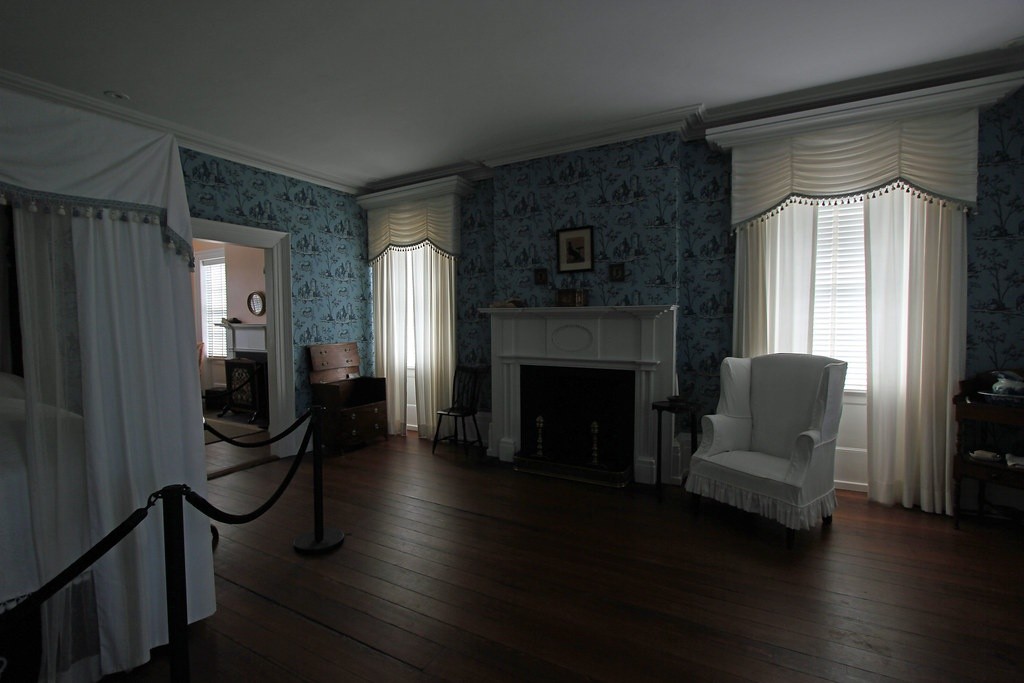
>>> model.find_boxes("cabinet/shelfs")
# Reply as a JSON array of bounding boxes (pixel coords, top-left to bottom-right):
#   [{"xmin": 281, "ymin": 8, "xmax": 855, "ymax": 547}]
[
  {"xmin": 952, "ymin": 395, "xmax": 1024, "ymax": 531},
  {"xmin": 307, "ymin": 343, "xmax": 388, "ymax": 456}
]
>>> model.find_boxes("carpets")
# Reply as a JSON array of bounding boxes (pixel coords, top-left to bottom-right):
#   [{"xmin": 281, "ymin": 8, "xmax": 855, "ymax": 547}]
[{"xmin": 203, "ymin": 409, "xmax": 266, "ymax": 445}]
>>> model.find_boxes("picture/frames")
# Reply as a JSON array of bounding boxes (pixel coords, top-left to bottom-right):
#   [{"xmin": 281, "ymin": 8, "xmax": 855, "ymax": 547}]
[
  {"xmin": 609, "ymin": 264, "xmax": 625, "ymax": 282},
  {"xmin": 534, "ymin": 268, "xmax": 547, "ymax": 285},
  {"xmin": 556, "ymin": 225, "xmax": 595, "ymax": 275}
]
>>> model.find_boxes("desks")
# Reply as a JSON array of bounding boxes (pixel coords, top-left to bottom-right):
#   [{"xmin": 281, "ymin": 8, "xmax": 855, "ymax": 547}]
[{"xmin": 651, "ymin": 401, "xmax": 714, "ymax": 506}]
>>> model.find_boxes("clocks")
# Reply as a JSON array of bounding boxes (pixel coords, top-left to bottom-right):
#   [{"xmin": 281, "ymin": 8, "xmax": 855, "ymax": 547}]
[{"xmin": 247, "ymin": 292, "xmax": 266, "ymax": 316}]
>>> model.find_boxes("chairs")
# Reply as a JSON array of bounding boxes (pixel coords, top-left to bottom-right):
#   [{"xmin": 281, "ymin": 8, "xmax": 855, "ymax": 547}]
[
  {"xmin": 432, "ymin": 368, "xmax": 484, "ymax": 457},
  {"xmin": 683, "ymin": 354, "xmax": 848, "ymax": 552}
]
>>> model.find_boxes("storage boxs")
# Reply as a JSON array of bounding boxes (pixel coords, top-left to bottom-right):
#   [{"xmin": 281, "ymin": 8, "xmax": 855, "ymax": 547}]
[
  {"xmin": 555, "ymin": 289, "xmax": 583, "ymax": 307},
  {"xmin": 206, "ymin": 387, "xmax": 226, "ymax": 410}
]
